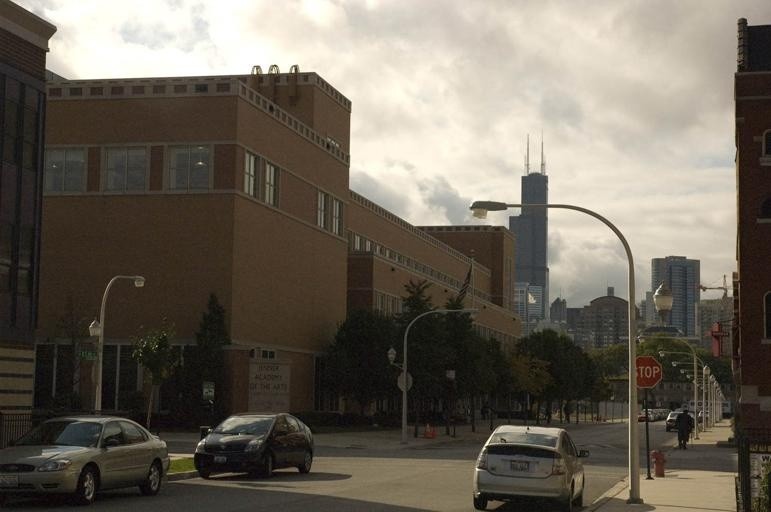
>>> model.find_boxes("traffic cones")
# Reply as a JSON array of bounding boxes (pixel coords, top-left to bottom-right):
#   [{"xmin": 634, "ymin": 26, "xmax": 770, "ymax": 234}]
[
  {"xmin": 423, "ymin": 421, "xmax": 429, "ymax": 438},
  {"xmin": 431, "ymin": 424, "xmax": 436, "ymax": 438}
]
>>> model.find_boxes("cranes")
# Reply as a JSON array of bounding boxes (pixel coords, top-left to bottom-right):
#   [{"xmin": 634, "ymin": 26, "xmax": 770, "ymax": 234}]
[{"xmin": 698, "ymin": 273, "xmax": 733, "ymax": 298}]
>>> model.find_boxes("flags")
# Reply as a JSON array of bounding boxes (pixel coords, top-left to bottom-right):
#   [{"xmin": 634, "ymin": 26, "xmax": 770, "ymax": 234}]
[{"xmin": 528, "ymin": 293, "xmax": 536, "ymax": 304}]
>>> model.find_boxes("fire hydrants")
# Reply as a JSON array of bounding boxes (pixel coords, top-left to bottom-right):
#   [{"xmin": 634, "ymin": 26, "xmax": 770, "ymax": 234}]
[{"xmin": 651, "ymin": 449, "xmax": 664, "ymax": 476}]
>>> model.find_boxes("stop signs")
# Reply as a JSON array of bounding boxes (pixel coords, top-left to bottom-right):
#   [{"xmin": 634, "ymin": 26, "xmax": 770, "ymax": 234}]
[{"xmin": 632, "ymin": 358, "xmax": 662, "ymax": 388}]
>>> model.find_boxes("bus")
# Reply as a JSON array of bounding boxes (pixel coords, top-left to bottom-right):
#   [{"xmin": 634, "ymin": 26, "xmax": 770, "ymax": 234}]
[{"xmin": 690, "ymin": 398, "xmax": 731, "ymax": 419}]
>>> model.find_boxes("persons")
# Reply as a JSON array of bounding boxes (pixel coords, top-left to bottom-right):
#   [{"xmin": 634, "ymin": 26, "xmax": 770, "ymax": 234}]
[
  {"xmin": 481, "ymin": 402, "xmax": 494, "ymax": 419},
  {"xmin": 675, "ymin": 410, "xmax": 692, "ymax": 449}
]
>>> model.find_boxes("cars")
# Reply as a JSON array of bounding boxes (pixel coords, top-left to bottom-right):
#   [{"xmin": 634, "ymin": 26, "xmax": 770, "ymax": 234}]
[
  {"xmin": 191, "ymin": 411, "xmax": 316, "ymax": 480},
  {"xmin": 1, "ymin": 414, "xmax": 170, "ymax": 505},
  {"xmin": 638, "ymin": 408, "xmax": 713, "ymax": 433},
  {"xmin": 473, "ymin": 419, "xmax": 590, "ymax": 511}
]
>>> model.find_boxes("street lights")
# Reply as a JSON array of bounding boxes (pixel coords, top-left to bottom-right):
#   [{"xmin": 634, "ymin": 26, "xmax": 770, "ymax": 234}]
[
  {"xmin": 468, "ymin": 200, "xmax": 641, "ymax": 505},
  {"xmin": 88, "ymin": 276, "xmax": 146, "ymax": 416},
  {"xmin": 443, "ymin": 362, "xmax": 456, "ymax": 436},
  {"xmin": 386, "ymin": 309, "xmax": 477, "ymax": 443},
  {"xmin": 637, "ymin": 335, "xmax": 725, "ymax": 441}
]
